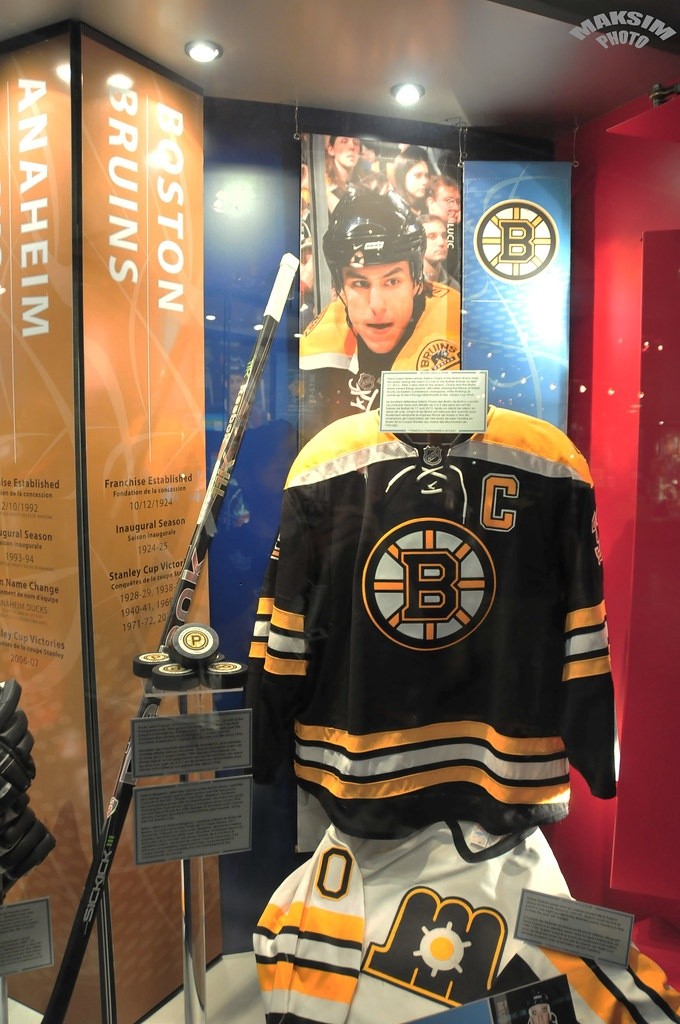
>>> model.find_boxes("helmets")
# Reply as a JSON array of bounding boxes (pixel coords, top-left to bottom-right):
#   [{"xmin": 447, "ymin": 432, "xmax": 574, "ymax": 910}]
[{"xmin": 323, "ymin": 175, "xmax": 427, "ymax": 296}]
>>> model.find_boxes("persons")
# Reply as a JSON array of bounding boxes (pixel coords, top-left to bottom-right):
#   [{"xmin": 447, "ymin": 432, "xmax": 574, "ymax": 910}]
[
  {"xmin": 300, "ymin": 134, "xmax": 460, "ymax": 450},
  {"xmin": 526, "ymin": 990, "xmax": 558, "ymax": 1024}
]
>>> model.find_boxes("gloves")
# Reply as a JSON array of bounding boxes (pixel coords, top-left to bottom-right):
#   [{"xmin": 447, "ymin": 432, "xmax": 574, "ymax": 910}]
[{"xmin": 0, "ymin": 674, "xmax": 57, "ymax": 910}]
